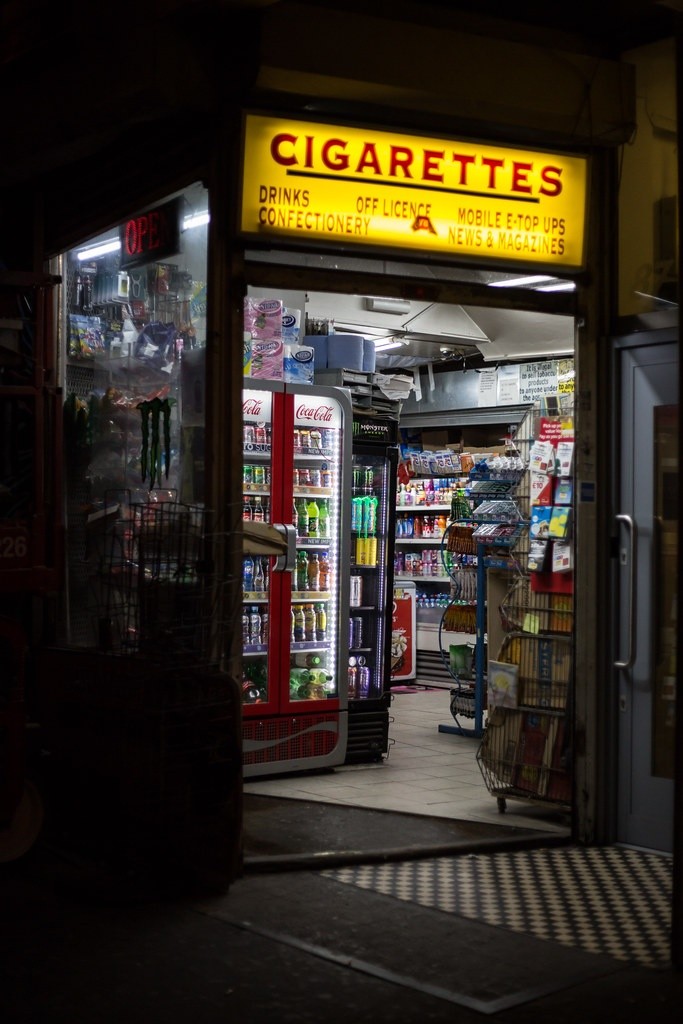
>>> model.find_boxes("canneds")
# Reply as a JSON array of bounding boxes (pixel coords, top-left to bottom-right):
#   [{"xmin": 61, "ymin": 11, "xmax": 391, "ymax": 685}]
[
  {"xmin": 348, "ymin": 655, "xmax": 370, "ymax": 700},
  {"xmin": 351, "ymin": 496, "xmax": 377, "ymax": 532},
  {"xmin": 243, "ymin": 426, "xmax": 271, "ymax": 445},
  {"xmin": 355, "ymin": 537, "xmax": 377, "ymax": 566},
  {"xmin": 349, "ymin": 575, "xmax": 363, "ymax": 607},
  {"xmin": 349, "ymin": 617, "xmax": 363, "ymax": 648},
  {"xmin": 352, "ymin": 466, "xmax": 362, "ymax": 487},
  {"xmin": 362, "ymin": 465, "xmax": 374, "ymax": 488},
  {"xmin": 243, "ymin": 466, "xmax": 270, "ymax": 485},
  {"xmin": 293, "ymin": 469, "xmax": 332, "ymax": 487}
]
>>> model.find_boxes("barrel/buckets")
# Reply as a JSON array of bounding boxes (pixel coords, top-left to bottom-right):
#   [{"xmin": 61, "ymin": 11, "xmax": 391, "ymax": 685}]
[{"xmin": 303, "ymin": 334, "xmax": 376, "ymax": 372}]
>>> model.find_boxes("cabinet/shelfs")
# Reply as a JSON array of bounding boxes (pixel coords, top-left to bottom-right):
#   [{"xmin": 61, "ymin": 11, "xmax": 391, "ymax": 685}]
[{"xmin": 236, "ymin": 373, "xmax": 578, "ymax": 830}]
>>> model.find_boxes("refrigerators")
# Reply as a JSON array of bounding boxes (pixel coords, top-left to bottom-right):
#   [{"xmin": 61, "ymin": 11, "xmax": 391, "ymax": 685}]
[
  {"xmin": 346, "ymin": 411, "xmax": 399, "ymax": 765},
  {"xmin": 242, "ymin": 371, "xmax": 348, "ymax": 777}
]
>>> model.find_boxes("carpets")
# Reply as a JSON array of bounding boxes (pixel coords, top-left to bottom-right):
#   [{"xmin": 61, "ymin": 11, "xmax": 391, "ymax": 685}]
[
  {"xmin": 184, "ymin": 867, "xmax": 629, "ymax": 1014},
  {"xmin": 237, "ymin": 785, "xmax": 560, "ymax": 857},
  {"xmin": 311, "ymin": 844, "xmax": 676, "ymax": 973}
]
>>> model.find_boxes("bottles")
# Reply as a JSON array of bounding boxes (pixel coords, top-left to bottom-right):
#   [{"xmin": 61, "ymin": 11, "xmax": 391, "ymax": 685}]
[
  {"xmin": 292, "ymin": 497, "xmax": 329, "ymax": 537},
  {"xmin": 242, "ymin": 556, "xmax": 269, "ymax": 592},
  {"xmin": 290, "ymin": 602, "xmax": 328, "ymax": 642},
  {"xmin": 292, "ymin": 548, "xmax": 330, "ymax": 592},
  {"xmin": 419, "ymin": 514, "xmax": 451, "ymax": 538},
  {"xmin": 244, "ymin": 495, "xmax": 270, "ymax": 523},
  {"xmin": 242, "ymin": 602, "xmax": 267, "ymax": 646},
  {"xmin": 289, "ymin": 652, "xmax": 333, "ymax": 699},
  {"xmin": 418, "ymin": 593, "xmax": 458, "ymax": 608}
]
